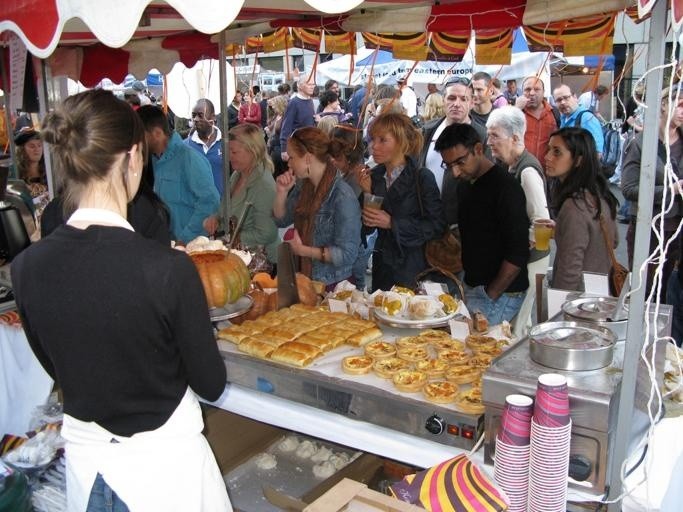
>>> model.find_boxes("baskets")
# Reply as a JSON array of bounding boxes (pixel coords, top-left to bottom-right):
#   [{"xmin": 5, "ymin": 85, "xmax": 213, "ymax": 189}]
[
  {"xmin": 228, "ymin": 215, "xmax": 268, "ymax": 278},
  {"xmin": 413, "ymin": 267, "xmax": 473, "ymax": 334}
]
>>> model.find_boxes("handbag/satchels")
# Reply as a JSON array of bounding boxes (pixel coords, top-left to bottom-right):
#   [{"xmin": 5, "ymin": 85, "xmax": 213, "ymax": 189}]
[
  {"xmin": 408, "ymin": 115, "xmax": 424, "ymax": 129},
  {"xmin": 624, "ymin": 124, "xmax": 636, "ymax": 154},
  {"xmin": 426, "ymin": 233, "xmax": 462, "ymax": 273},
  {"xmin": 600, "ymin": 123, "xmax": 621, "ymax": 177},
  {"xmin": 610, "ymin": 262, "xmax": 627, "ymax": 297}
]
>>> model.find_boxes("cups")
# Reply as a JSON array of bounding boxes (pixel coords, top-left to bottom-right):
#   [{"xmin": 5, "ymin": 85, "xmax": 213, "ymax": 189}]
[
  {"xmin": 363, "ymin": 192, "xmax": 384, "ymax": 226},
  {"xmin": 526, "ymin": 372, "xmax": 573, "ymax": 512},
  {"xmin": 533, "ymin": 218, "xmax": 555, "ymax": 250},
  {"xmin": 494, "ymin": 393, "xmax": 533, "ymax": 512}
]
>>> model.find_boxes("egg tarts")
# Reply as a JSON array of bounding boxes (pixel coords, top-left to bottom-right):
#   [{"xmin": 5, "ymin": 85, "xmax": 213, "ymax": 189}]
[{"xmin": 342, "ymin": 329, "xmax": 504, "ymax": 413}]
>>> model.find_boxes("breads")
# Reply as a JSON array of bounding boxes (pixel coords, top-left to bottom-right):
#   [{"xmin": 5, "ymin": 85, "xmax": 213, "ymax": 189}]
[
  {"xmin": 374, "ymin": 287, "xmax": 457, "ymax": 319},
  {"xmin": 218, "ymin": 288, "xmax": 382, "ymax": 368}
]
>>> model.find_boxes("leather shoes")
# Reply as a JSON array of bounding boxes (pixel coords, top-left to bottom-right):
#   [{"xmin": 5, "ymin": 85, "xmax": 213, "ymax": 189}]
[{"xmin": 616, "ymin": 216, "xmax": 630, "ymax": 223}]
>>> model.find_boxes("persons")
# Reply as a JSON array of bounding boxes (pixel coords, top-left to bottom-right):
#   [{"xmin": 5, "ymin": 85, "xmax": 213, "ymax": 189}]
[
  {"xmin": 1, "ymin": 63, "xmax": 683, "ymax": 347},
  {"xmin": 8, "ymin": 87, "xmax": 238, "ymax": 512}
]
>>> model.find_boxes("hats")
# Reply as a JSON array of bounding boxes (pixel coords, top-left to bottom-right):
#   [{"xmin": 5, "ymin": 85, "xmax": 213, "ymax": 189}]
[
  {"xmin": 635, "ymin": 86, "xmax": 646, "ymax": 95},
  {"xmin": 14, "ymin": 131, "xmax": 41, "ymax": 145},
  {"xmin": 329, "ymin": 126, "xmax": 364, "ymax": 164}
]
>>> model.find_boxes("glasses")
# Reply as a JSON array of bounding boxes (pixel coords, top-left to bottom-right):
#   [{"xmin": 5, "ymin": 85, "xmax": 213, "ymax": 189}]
[
  {"xmin": 488, "ymin": 133, "xmax": 504, "ymax": 142},
  {"xmin": 556, "ymin": 95, "xmax": 572, "ymax": 104},
  {"xmin": 446, "ymin": 78, "xmax": 472, "ymax": 86},
  {"xmin": 440, "ymin": 147, "xmax": 471, "ymax": 169},
  {"xmin": 289, "ymin": 128, "xmax": 313, "ymax": 154}
]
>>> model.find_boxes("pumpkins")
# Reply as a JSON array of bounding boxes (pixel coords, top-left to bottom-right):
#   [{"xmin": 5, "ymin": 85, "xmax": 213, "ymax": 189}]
[{"xmin": 189, "ymin": 249, "xmax": 251, "ymax": 310}]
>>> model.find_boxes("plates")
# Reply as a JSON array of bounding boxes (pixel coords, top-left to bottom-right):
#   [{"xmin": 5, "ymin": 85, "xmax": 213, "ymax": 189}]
[{"xmin": 371, "ymin": 307, "xmax": 461, "ymax": 331}]
